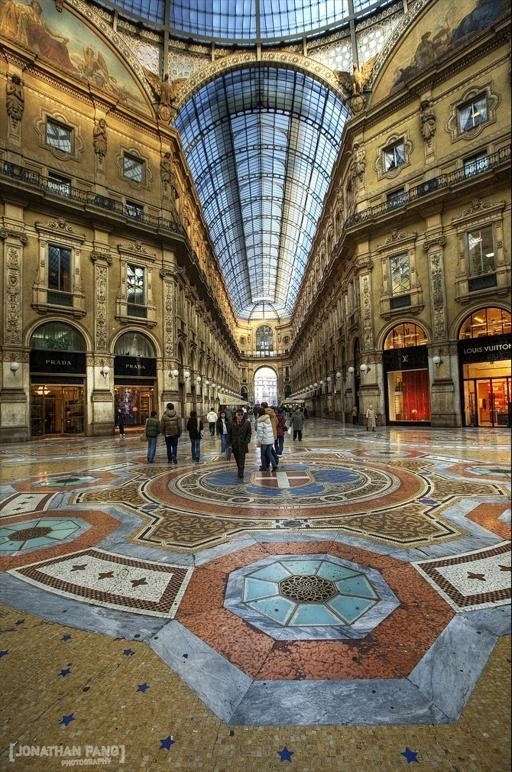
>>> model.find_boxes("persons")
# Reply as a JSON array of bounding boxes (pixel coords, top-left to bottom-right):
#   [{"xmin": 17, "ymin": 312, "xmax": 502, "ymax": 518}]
[
  {"xmin": 115, "ymin": 407, "xmax": 128, "ymax": 438},
  {"xmin": 205, "ymin": 400, "xmax": 306, "ymax": 478},
  {"xmin": 186, "ymin": 410, "xmax": 203, "ymax": 462},
  {"xmin": 144, "ymin": 410, "xmax": 160, "ymax": 463},
  {"xmin": 350, "ymin": 404, "xmax": 358, "ymax": 426},
  {"xmin": 365, "ymin": 405, "xmax": 379, "ymax": 432},
  {"xmin": 160, "ymin": 403, "xmax": 183, "ymax": 465}
]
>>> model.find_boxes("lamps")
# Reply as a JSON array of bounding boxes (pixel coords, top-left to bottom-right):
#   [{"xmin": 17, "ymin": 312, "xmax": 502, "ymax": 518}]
[
  {"xmin": 360, "ymin": 364, "xmax": 371, "ymax": 372},
  {"xmin": 348, "ymin": 367, "xmax": 355, "ymax": 375},
  {"xmin": 100, "ymin": 366, "xmax": 110, "ymax": 378},
  {"xmin": 300, "ymin": 372, "xmax": 341, "ymax": 393},
  {"xmin": 10, "ymin": 362, "xmax": 20, "ymax": 376},
  {"xmin": 433, "ymin": 355, "xmax": 444, "ymax": 369},
  {"xmin": 168, "ymin": 370, "xmax": 234, "ymax": 394}
]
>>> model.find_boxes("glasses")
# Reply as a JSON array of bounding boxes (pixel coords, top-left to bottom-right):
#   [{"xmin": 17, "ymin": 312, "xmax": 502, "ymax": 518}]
[{"xmin": 238, "ymin": 413, "xmax": 244, "ymax": 417}]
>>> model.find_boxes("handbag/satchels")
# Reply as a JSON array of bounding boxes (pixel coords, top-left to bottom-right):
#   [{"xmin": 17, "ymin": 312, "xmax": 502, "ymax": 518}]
[
  {"xmin": 282, "ymin": 426, "xmax": 287, "ymax": 432},
  {"xmin": 140, "ymin": 433, "xmax": 148, "ymax": 441}
]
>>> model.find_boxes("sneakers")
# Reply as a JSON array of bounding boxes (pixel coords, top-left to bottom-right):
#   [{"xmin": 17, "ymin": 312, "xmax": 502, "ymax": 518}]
[
  {"xmin": 191, "ymin": 456, "xmax": 200, "ymax": 462},
  {"xmin": 239, "ymin": 472, "xmax": 243, "ymax": 478},
  {"xmin": 167, "ymin": 459, "xmax": 177, "ymax": 463},
  {"xmin": 259, "ymin": 464, "xmax": 277, "ymax": 472},
  {"xmin": 276, "ymin": 454, "xmax": 283, "ymax": 457},
  {"xmin": 146, "ymin": 457, "xmax": 155, "ymax": 463}
]
